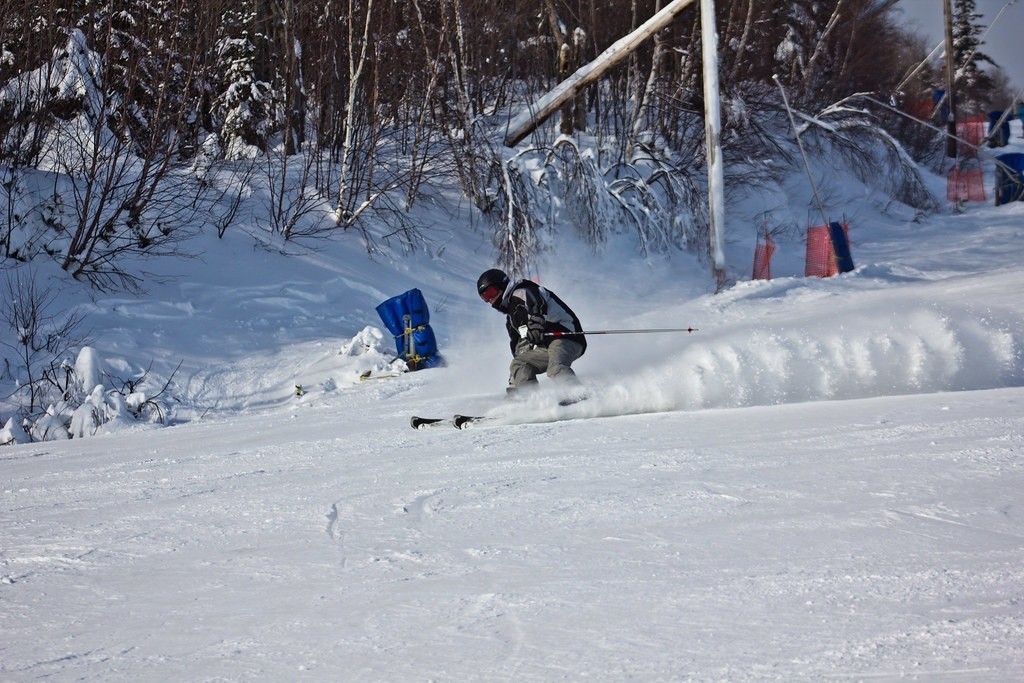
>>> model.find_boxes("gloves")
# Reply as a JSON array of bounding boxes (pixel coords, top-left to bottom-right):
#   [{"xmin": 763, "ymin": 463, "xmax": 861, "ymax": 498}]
[{"xmin": 526, "ymin": 314, "xmax": 545, "ymax": 345}]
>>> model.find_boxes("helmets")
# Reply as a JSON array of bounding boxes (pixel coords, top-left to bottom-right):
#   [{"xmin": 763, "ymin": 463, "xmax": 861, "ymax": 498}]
[{"xmin": 477, "ymin": 269, "xmax": 510, "ymax": 301}]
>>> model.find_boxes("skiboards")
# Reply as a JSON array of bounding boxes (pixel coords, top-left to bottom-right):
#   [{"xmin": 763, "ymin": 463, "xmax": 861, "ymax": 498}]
[{"xmin": 410, "ymin": 414, "xmax": 488, "ymax": 431}]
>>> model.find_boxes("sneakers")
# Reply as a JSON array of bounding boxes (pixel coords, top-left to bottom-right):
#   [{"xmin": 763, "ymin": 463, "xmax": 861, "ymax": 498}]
[{"xmin": 559, "ymin": 391, "xmax": 591, "ymax": 407}]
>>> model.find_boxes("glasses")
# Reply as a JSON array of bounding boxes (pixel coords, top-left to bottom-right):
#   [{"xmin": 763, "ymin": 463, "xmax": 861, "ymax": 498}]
[{"xmin": 481, "ymin": 287, "xmax": 498, "ymax": 302}]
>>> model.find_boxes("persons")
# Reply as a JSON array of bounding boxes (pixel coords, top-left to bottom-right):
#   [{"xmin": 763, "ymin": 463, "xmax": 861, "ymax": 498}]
[
  {"xmin": 477, "ymin": 269, "xmax": 587, "ymax": 405},
  {"xmin": 1014, "ymin": 102, "xmax": 1024, "ymax": 138}
]
